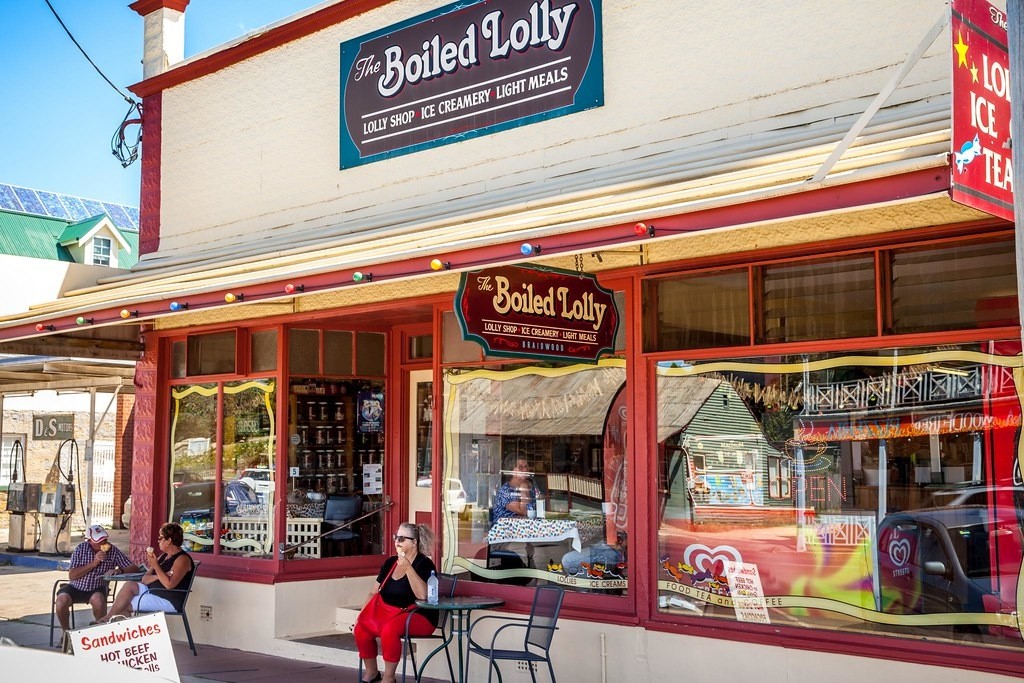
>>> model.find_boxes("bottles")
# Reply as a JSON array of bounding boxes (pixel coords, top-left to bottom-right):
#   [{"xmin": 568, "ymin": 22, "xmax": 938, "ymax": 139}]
[{"xmin": 427, "ymin": 570, "xmax": 439, "ymax": 603}]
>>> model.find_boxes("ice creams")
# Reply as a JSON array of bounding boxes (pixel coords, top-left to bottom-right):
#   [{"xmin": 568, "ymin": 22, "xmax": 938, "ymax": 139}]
[
  {"xmin": 146, "ymin": 547, "xmax": 154, "ymax": 557},
  {"xmin": 397, "ymin": 547, "xmax": 405, "ymax": 557}
]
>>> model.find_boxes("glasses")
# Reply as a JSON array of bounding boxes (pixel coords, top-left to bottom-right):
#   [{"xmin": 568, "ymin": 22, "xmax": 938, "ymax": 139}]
[
  {"xmin": 158, "ymin": 536, "xmax": 167, "ymax": 540},
  {"xmin": 514, "ymin": 464, "xmax": 529, "ymax": 469},
  {"xmin": 392, "ymin": 535, "xmax": 414, "ymax": 542}
]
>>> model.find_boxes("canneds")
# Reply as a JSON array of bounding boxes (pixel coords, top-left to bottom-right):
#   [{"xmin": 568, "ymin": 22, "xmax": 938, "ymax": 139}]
[{"xmin": 295, "ymin": 383, "xmax": 384, "ymax": 493}]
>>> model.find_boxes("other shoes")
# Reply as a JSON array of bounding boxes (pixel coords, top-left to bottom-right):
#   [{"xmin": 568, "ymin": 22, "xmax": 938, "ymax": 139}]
[
  {"xmin": 55, "ymin": 637, "xmax": 63, "ymax": 647},
  {"xmin": 361, "ymin": 671, "xmax": 382, "ymax": 683},
  {"xmin": 89, "ymin": 619, "xmax": 106, "ymax": 625}
]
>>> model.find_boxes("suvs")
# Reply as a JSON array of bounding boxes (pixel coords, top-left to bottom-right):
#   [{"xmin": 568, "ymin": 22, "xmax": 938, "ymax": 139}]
[{"xmin": 230, "ymin": 466, "xmax": 276, "ymax": 505}]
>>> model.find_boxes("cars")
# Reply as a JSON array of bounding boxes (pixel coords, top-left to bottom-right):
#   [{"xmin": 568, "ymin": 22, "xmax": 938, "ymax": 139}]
[
  {"xmin": 876, "ymin": 485, "xmax": 1024, "ymax": 646},
  {"xmin": 172, "ymin": 478, "xmax": 261, "ymax": 525},
  {"xmin": 173, "ymin": 470, "xmax": 204, "ymax": 488}
]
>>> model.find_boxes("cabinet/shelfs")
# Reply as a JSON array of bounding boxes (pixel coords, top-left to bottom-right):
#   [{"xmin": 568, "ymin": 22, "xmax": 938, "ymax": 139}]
[{"xmin": 859, "ymin": 484, "xmax": 963, "ymax": 512}]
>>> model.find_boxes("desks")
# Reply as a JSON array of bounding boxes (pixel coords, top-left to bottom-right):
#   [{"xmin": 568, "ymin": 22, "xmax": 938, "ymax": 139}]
[
  {"xmin": 487, "ymin": 515, "xmax": 606, "ymax": 552},
  {"xmin": 415, "ymin": 595, "xmax": 506, "ymax": 683},
  {"xmin": 104, "ymin": 573, "xmax": 148, "ymax": 581}
]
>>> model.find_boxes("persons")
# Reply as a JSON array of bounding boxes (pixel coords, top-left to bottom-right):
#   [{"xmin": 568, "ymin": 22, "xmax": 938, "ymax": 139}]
[
  {"xmin": 490, "ymin": 449, "xmax": 542, "ymax": 569},
  {"xmin": 353, "ymin": 522, "xmax": 439, "ymax": 682},
  {"xmin": 89, "ymin": 522, "xmax": 195, "ymax": 625},
  {"xmin": 56, "ymin": 525, "xmax": 139, "ymax": 648},
  {"xmin": 561, "ymin": 531, "xmax": 628, "ymax": 594}
]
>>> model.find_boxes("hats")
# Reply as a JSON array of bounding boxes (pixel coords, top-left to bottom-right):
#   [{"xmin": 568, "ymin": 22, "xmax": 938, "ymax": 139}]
[{"xmin": 85, "ymin": 525, "xmax": 109, "ymax": 543}]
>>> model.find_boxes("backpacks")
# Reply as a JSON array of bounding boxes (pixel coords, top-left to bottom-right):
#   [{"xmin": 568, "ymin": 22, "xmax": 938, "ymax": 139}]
[{"xmin": 357, "ymin": 592, "xmax": 407, "ymax": 636}]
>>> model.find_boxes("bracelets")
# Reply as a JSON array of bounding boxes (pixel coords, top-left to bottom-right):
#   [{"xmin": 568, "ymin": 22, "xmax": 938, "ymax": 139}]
[{"xmin": 118, "ymin": 566, "xmax": 124, "ymax": 573}]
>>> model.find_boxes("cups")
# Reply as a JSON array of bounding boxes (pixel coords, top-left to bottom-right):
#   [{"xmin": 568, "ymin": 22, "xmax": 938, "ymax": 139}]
[
  {"xmin": 314, "ymin": 493, "xmax": 323, "ymax": 500},
  {"xmin": 570, "ymin": 509, "xmax": 582, "ymax": 517},
  {"xmin": 601, "ymin": 502, "xmax": 618, "ymax": 514},
  {"xmin": 527, "ymin": 510, "xmax": 536, "ymax": 519}
]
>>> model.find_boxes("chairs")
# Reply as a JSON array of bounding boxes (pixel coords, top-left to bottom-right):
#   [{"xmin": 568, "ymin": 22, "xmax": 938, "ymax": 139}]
[
  {"xmin": 465, "ymin": 585, "xmax": 565, "ymax": 683},
  {"xmin": 486, "ymin": 508, "xmax": 496, "ymax": 570},
  {"xmin": 49, "ymin": 576, "xmax": 118, "ymax": 646},
  {"xmin": 133, "ymin": 560, "xmax": 200, "ymax": 656},
  {"xmin": 927, "ymin": 532, "xmax": 969, "ymax": 580},
  {"xmin": 359, "ymin": 573, "xmax": 458, "ymax": 683}
]
